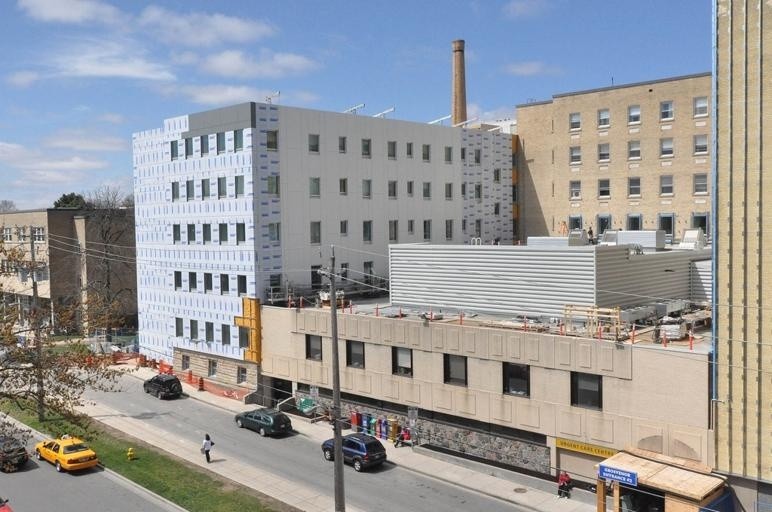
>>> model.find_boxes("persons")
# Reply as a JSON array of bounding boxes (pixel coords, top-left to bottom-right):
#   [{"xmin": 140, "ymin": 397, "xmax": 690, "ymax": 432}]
[
  {"xmin": 556, "ymin": 470, "xmax": 570, "ymax": 499},
  {"xmin": 393, "ymin": 427, "xmax": 409, "ymax": 447},
  {"xmin": 587, "ymin": 226, "xmax": 594, "ymax": 245},
  {"xmin": 202, "ymin": 434, "xmax": 212, "ymax": 462}
]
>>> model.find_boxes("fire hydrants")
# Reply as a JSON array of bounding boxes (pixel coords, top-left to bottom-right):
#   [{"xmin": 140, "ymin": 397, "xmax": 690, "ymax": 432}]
[{"xmin": 127, "ymin": 446, "xmax": 134, "ymax": 461}]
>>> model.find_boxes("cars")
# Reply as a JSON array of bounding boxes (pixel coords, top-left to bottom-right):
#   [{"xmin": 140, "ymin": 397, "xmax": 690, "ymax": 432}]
[
  {"xmin": 0, "ymin": 437, "xmax": 29, "ymax": 470},
  {"xmin": 35, "ymin": 433, "xmax": 98, "ymax": 472},
  {"xmin": 0, "ymin": 496, "xmax": 12, "ymax": 512},
  {"xmin": 234, "ymin": 407, "xmax": 292, "ymax": 439}
]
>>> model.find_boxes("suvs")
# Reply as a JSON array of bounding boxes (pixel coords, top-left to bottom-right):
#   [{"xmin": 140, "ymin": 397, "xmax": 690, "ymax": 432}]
[
  {"xmin": 143, "ymin": 373, "xmax": 184, "ymax": 400},
  {"xmin": 321, "ymin": 432, "xmax": 387, "ymax": 472}
]
